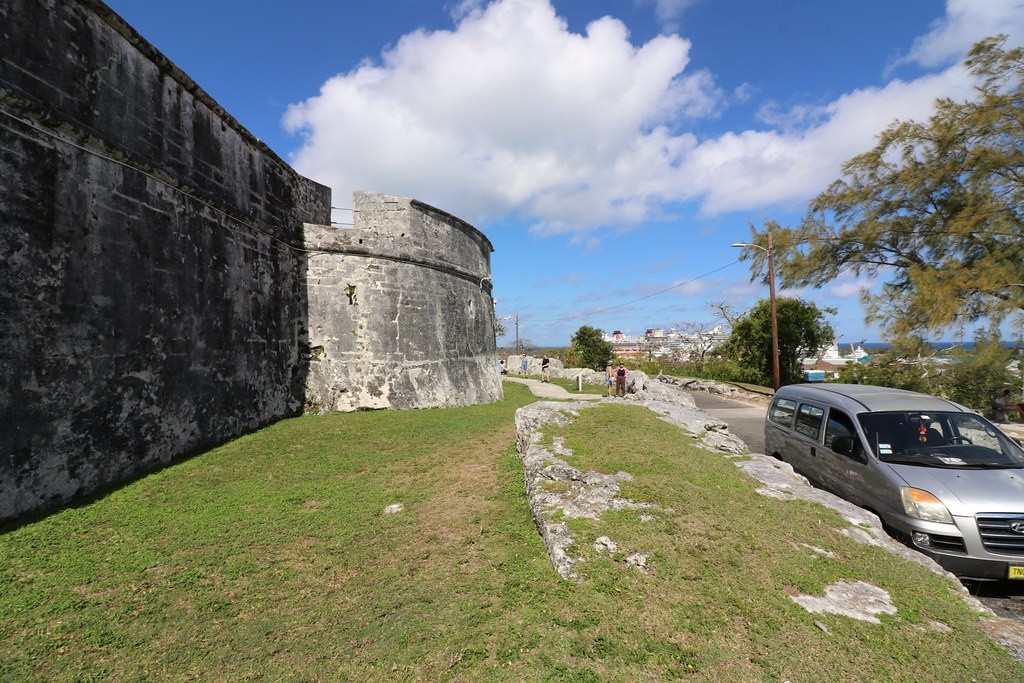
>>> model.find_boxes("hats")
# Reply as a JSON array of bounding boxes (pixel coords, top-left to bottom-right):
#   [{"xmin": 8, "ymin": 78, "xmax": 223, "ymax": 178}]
[
  {"xmin": 607, "ymin": 361, "xmax": 613, "ymax": 365},
  {"xmin": 619, "ymin": 362, "xmax": 624, "ymax": 364}
]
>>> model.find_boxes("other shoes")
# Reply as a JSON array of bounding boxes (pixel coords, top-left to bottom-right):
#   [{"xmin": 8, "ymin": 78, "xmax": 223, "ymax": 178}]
[{"xmin": 541, "ymin": 379, "xmax": 550, "ymax": 384}]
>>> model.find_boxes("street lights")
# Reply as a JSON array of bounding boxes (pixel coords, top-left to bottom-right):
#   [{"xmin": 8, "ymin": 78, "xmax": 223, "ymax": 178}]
[
  {"xmin": 731, "ymin": 243, "xmax": 780, "ymax": 394},
  {"xmin": 503, "ymin": 318, "xmax": 519, "ymax": 356}
]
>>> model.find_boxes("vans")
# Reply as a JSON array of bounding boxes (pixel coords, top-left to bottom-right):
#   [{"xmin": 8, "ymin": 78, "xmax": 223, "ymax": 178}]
[{"xmin": 764, "ymin": 383, "xmax": 1024, "ymax": 590}]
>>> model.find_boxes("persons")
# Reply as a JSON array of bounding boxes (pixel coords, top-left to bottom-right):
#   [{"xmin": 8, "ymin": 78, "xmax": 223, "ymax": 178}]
[
  {"xmin": 522, "ymin": 354, "xmax": 527, "ymax": 377},
  {"xmin": 606, "ymin": 360, "xmax": 630, "ymax": 397},
  {"xmin": 500, "ymin": 361, "xmax": 507, "ymax": 374},
  {"xmin": 542, "ymin": 355, "xmax": 549, "ymax": 382}
]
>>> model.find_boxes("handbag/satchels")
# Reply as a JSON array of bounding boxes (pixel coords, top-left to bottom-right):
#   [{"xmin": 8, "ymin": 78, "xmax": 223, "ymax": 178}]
[{"xmin": 607, "ymin": 379, "xmax": 612, "ymax": 384}]
